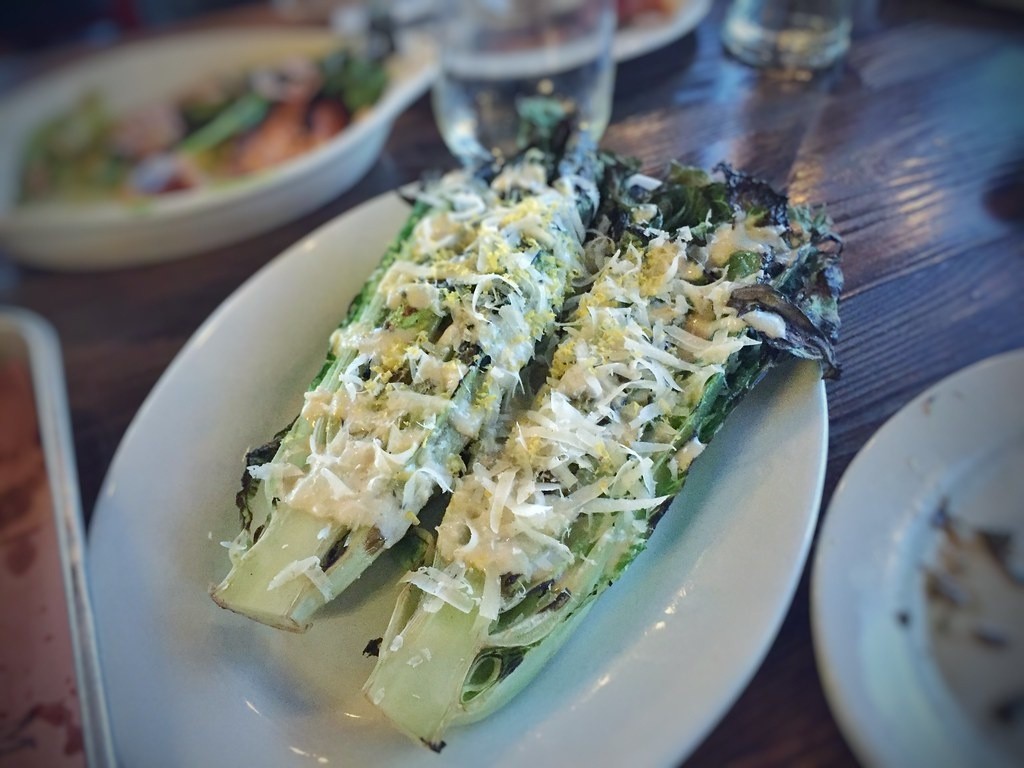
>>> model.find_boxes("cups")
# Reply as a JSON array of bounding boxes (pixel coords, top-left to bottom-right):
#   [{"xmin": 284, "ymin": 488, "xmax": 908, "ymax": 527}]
[{"xmin": 419, "ymin": 1, "xmax": 620, "ymax": 169}]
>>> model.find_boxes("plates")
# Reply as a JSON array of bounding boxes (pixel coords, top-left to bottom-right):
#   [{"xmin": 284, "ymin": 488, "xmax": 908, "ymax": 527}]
[
  {"xmin": 0, "ymin": 23, "xmax": 436, "ymax": 268},
  {"xmin": 330, "ymin": 0, "xmax": 714, "ymax": 81},
  {"xmin": 808, "ymin": 349, "xmax": 1024, "ymax": 768},
  {"xmin": 89, "ymin": 162, "xmax": 826, "ymax": 768}
]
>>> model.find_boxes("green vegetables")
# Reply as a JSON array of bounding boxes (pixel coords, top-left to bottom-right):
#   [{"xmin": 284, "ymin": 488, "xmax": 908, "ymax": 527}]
[{"xmin": 204, "ymin": 101, "xmax": 843, "ymax": 754}]
